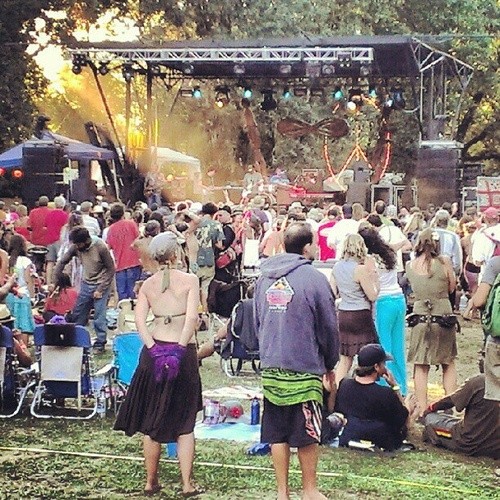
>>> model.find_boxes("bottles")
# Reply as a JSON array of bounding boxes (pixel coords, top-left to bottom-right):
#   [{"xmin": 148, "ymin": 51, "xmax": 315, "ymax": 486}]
[
  {"xmin": 250, "ymin": 396, "xmax": 260, "ymax": 425},
  {"xmin": 99, "ymin": 389, "xmax": 107, "ymax": 419}
]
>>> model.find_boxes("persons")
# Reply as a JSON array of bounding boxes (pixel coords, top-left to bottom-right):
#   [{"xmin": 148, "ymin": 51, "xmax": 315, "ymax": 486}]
[
  {"xmin": 143, "ymin": 164, "xmax": 288, "ymax": 206},
  {"xmin": 0, "ymin": 188, "xmax": 500, "ymax": 500}
]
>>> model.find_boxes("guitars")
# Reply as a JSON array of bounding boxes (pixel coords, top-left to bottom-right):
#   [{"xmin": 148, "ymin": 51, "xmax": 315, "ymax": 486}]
[
  {"xmin": 143, "ymin": 174, "xmax": 174, "ymax": 197},
  {"xmin": 202, "ymin": 185, "xmax": 232, "ymax": 195},
  {"xmin": 242, "ymin": 179, "xmax": 264, "ymax": 198}
]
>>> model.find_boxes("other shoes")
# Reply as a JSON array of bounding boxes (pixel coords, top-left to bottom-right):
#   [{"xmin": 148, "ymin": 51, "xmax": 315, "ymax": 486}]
[
  {"xmin": 143, "ymin": 481, "xmax": 164, "ymax": 495},
  {"xmin": 92, "ymin": 341, "xmax": 107, "ymax": 355},
  {"xmin": 182, "ymin": 483, "xmax": 207, "ymax": 497}
]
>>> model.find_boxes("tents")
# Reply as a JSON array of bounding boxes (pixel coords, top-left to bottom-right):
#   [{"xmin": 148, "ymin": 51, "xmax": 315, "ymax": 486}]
[{"xmin": 0, "ymin": 130, "xmax": 206, "ymax": 205}]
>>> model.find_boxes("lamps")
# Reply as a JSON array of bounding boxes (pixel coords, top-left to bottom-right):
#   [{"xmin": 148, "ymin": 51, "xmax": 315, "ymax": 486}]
[{"xmin": 72, "ymin": 53, "xmax": 406, "ymax": 112}]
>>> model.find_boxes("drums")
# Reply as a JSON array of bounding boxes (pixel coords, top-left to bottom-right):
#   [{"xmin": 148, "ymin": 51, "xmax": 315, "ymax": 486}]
[
  {"xmin": 241, "ymin": 193, "xmax": 272, "ymax": 210},
  {"xmin": 263, "ymin": 184, "xmax": 277, "ymax": 191}
]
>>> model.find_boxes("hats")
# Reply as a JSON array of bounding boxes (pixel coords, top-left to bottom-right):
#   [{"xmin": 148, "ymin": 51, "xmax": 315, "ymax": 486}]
[
  {"xmin": 250, "ymin": 195, "xmax": 266, "ymax": 208},
  {"xmin": 144, "ymin": 203, "xmax": 171, "ymax": 220},
  {"xmin": 358, "ymin": 344, "xmax": 393, "ymax": 367},
  {"xmin": 0, "ymin": 200, "xmax": 7, "ymax": 205},
  {"xmin": 220, "ymin": 204, "xmax": 246, "ymax": 218},
  {"xmin": 80, "ymin": 195, "xmax": 111, "ymax": 213},
  {"xmin": 289, "ymin": 202, "xmax": 305, "ymax": 212},
  {"xmin": 484, "ymin": 222, "xmax": 500, "ymax": 243},
  {"xmin": 0, "ymin": 304, "xmax": 16, "ymax": 323},
  {"xmin": 148, "ymin": 231, "xmax": 186, "ymax": 262},
  {"xmin": 39, "ymin": 196, "xmax": 50, "ymax": 203},
  {"xmin": 485, "ymin": 207, "xmax": 498, "ymax": 219},
  {"xmin": 467, "ymin": 207, "xmax": 477, "ymax": 215},
  {"xmin": 442, "ymin": 202, "xmax": 453, "ymax": 209}
]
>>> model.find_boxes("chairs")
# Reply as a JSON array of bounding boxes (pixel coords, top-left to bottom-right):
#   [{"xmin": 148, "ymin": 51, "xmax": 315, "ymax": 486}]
[
  {"xmin": 218, "ymin": 298, "xmax": 261, "ymax": 380},
  {"xmin": 115, "ymin": 298, "xmax": 153, "ymax": 333},
  {"xmin": 31, "ymin": 324, "xmax": 113, "ymax": 418},
  {"xmin": 0, "ymin": 323, "xmax": 39, "ymax": 420},
  {"xmin": 108, "ymin": 332, "xmax": 146, "ymax": 417}
]
